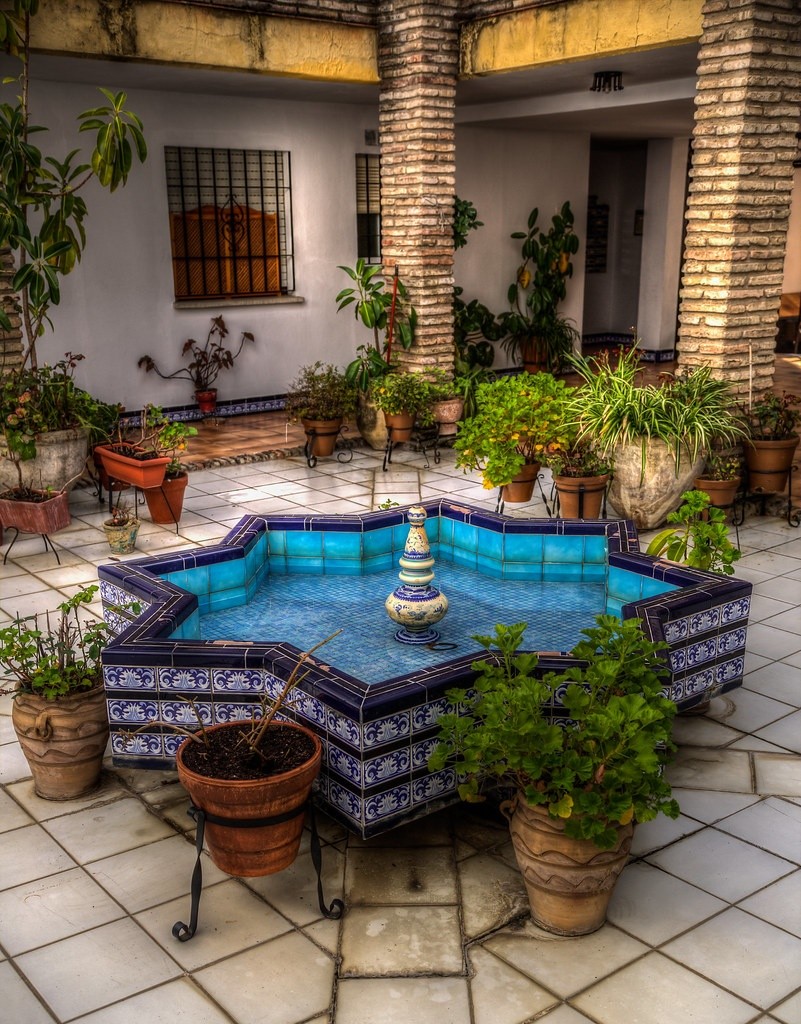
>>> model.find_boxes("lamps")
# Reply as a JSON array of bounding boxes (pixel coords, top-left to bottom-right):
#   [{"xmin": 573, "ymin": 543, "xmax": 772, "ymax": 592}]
[{"xmin": 590, "ymin": 71, "xmax": 624, "ymax": 93}]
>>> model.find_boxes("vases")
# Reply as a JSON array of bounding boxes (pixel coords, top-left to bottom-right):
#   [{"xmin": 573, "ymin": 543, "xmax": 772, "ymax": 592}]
[{"xmin": 177, "ymin": 720, "xmax": 324, "ymax": 877}]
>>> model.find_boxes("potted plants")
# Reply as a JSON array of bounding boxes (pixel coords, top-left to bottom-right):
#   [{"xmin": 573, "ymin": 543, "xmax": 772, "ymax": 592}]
[{"xmin": 0, "ymin": 0, "xmax": 801, "ymax": 939}]
